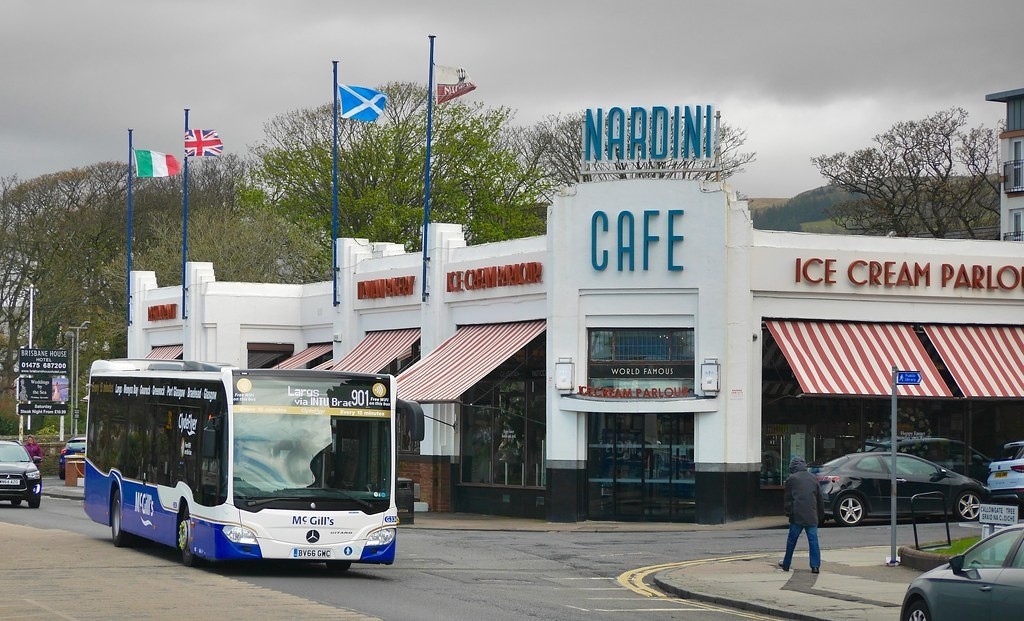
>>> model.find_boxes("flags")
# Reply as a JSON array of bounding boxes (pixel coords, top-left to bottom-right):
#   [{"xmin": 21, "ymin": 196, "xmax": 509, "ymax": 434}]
[
  {"xmin": 338, "ymin": 84, "xmax": 388, "ymax": 121},
  {"xmin": 434, "ymin": 64, "xmax": 477, "ymax": 106},
  {"xmin": 133, "ymin": 150, "xmax": 180, "ymax": 177},
  {"xmin": 184, "ymin": 129, "xmax": 224, "ymax": 156}
]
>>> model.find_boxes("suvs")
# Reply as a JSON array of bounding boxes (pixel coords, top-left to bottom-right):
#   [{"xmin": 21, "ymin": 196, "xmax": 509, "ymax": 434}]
[
  {"xmin": 985, "ymin": 440, "xmax": 1024, "ymax": 517},
  {"xmin": 0, "ymin": 440, "xmax": 42, "ymax": 509},
  {"xmin": 856, "ymin": 435, "xmax": 994, "ymax": 484},
  {"xmin": 59, "ymin": 435, "xmax": 86, "ymax": 480}
]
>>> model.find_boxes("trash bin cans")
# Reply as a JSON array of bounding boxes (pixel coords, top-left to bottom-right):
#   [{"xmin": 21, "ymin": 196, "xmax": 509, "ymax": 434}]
[{"xmin": 398, "ymin": 477, "xmax": 414, "ymax": 524}]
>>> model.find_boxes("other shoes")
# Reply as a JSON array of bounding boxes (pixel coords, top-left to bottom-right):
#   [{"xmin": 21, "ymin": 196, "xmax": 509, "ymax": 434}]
[
  {"xmin": 812, "ymin": 567, "xmax": 819, "ymax": 573},
  {"xmin": 779, "ymin": 560, "xmax": 789, "ymax": 571}
]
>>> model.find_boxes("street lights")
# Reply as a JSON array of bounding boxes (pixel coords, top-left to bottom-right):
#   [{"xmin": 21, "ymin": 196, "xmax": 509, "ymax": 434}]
[
  {"xmin": 66, "ymin": 331, "xmax": 75, "ymax": 434},
  {"xmin": 74, "ymin": 319, "xmax": 91, "ymax": 434}
]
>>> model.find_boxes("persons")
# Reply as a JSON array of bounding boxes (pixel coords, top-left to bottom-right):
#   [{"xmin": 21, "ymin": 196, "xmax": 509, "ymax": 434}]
[
  {"xmin": 779, "ymin": 456, "xmax": 825, "ymax": 573},
  {"xmin": 23, "ymin": 434, "xmax": 42, "ymax": 468}
]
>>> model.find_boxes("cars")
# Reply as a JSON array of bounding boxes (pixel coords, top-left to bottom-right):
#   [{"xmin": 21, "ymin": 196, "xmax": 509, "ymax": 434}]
[
  {"xmin": 800, "ymin": 450, "xmax": 988, "ymax": 527},
  {"xmin": 898, "ymin": 524, "xmax": 1023, "ymax": 621}
]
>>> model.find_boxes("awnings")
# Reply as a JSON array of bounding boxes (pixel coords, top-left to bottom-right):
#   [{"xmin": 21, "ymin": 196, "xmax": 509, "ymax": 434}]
[
  {"xmin": 271, "ymin": 327, "xmax": 421, "ymax": 374},
  {"xmin": 394, "ymin": 319, "xmax": 546, "ymax": 403},
  {"xmin": 145, "ymin": 345, "xmax": 183, "ymax": 359},
  {"xmin": 248, "ymin": 352, "xmax": 284, "ymax": 368},
  {"xmin": 764, "ymin": 320, "xmax": 1024, "ymax": 401}
]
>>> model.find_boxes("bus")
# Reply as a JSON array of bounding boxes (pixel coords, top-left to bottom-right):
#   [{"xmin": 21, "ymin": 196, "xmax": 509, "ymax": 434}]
[{"xmin": 83, "ymin": 357, "xmax": 425, "ymax": 573}]
